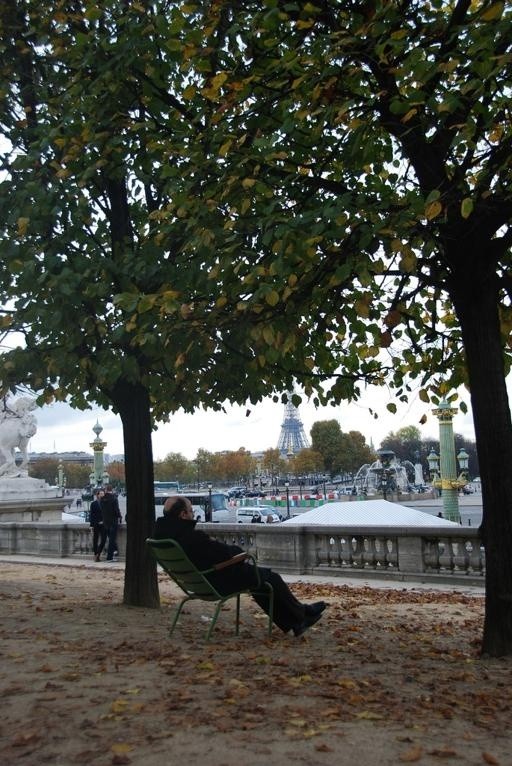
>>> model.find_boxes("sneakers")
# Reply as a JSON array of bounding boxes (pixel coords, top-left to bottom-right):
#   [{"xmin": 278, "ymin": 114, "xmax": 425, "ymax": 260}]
[{"xmin": 94, "ymin": 550, "xmax": 118, "ymax": 562}]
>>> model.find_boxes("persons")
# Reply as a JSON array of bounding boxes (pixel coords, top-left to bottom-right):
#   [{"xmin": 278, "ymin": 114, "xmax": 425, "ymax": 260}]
[
  {"xmin": 267, "ymin": 516, "xmax": 273, "ymax": 522},
  {"xmin": 90, "ymin": 490, "xmax": 104, "ymax": 562},
  {"xmin": 101, "ymin": 485, "xmax": 122, "ymax": 556},
  {"xmin": 152, "ymin": 494, "xmax": 326, "ymax": 637},
  {"xmin": 258, "ymin": 516, "xmax": 261, "ymax": 522},
  {"xmin": 251, "ymin": 515, "xmax": 257, "ymax": 523},
  {"xmin": 437, "ymin": 512, "xmax": 442, "ymax": 518}
]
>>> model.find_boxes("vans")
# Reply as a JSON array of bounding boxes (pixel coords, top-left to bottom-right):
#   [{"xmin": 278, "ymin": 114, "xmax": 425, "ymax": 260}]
[{"xmin": 237, "ymin": 505, "xmax": 282, "ymax": 524}]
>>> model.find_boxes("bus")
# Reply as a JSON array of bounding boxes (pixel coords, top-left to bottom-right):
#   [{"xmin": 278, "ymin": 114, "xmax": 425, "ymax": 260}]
[{"xmin": 155, "ymin": 492, "xmax": 231, "ymax": 523}]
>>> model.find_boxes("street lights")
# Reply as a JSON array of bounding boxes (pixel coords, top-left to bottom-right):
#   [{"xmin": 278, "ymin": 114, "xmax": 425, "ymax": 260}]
[
  {"xmin": 102, "ymin": 471, "xmax": 110, "ymax": 485},
  {"xmin": 299, "ymin": 477, "xmax": 303, "ymax": 495},
  {"xmin": 382, "ymin": 472, "xmax": 388, "ymax": 500},
  {"xmin": 323, "ymin": 474, "xmax": 326, "ymax": 495},
  {"xmin": 192, "ymin": 460, "xmax": 200, "ymax": 491},
  {"xmin": 207, "ymin": 482, "xmax": 212, "ymax": 522},
  {"xmin": 284, "ymin": 477, "xmax": 290, "ymax": 514}
]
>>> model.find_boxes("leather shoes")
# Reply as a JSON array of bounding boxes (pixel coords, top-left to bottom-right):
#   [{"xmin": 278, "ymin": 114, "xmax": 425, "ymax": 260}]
[{"xmin": 294, "ymin": 601, "xmax": 326, "ymax": 636}]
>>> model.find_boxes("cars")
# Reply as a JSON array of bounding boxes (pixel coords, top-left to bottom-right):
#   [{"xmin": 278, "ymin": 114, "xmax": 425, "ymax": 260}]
[
  {"xmin": 228, "ymin": 487, "xmax": 267, "ymax": 498},
  {"xmin": 69, "ymin": 510, "xmax": 91, "ymax": 522}
]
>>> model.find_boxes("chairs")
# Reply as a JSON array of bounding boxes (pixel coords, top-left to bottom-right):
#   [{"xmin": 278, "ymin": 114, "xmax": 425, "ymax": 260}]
[{"xmin": 143, "ymin": 537, "xmax": 275, "ymax": 643}]
